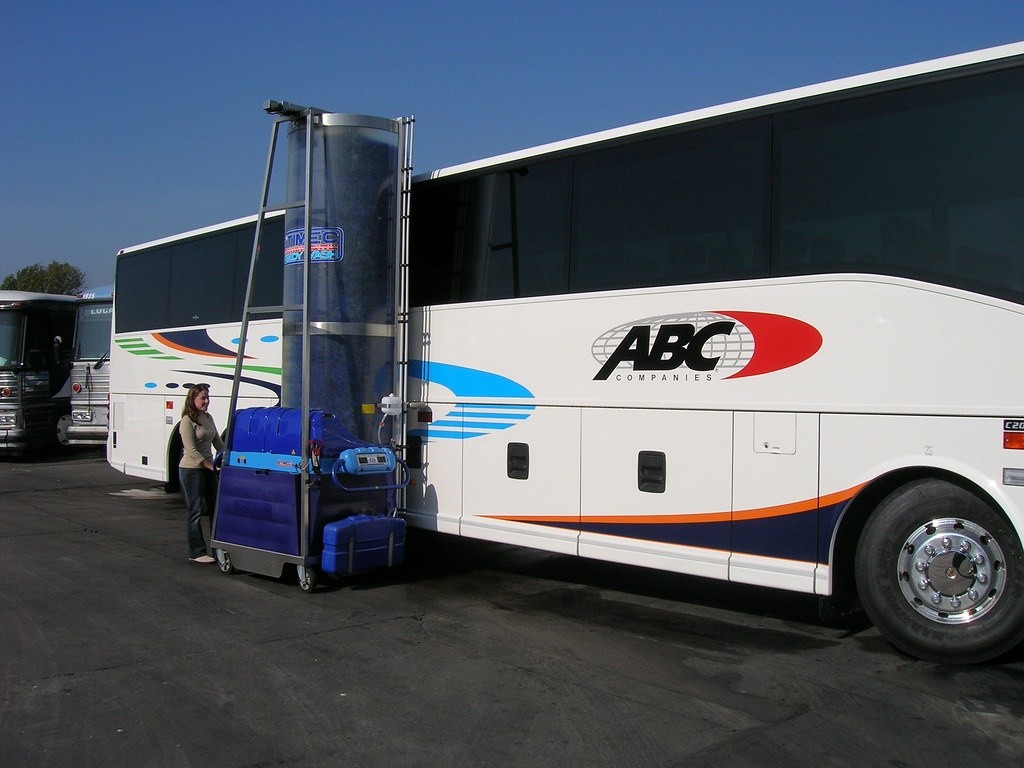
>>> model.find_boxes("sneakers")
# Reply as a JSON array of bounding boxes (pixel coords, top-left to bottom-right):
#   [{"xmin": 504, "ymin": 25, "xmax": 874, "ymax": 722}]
[{"xmin": 188, "ymin": 553, "xmax": 216, "ymax": 564}]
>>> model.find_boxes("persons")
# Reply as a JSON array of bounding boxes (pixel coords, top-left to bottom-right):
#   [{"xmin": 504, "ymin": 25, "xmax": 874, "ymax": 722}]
[{"xmin": 178, "ymin": 384, "xmax": 227, "ymax": 564}]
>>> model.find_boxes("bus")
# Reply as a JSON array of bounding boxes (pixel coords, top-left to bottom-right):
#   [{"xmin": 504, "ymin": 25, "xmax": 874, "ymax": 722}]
[
  {"xmin": 106, "ymin": 36, "xmax": 1024, "ymax": 661},
  {"xmin": 53, "ymin": 284, "xmax": 109, "ymax": 446},
  {"xmin": 0, "ymin": 290, "xmax": 80, "ymax": 449}
]
